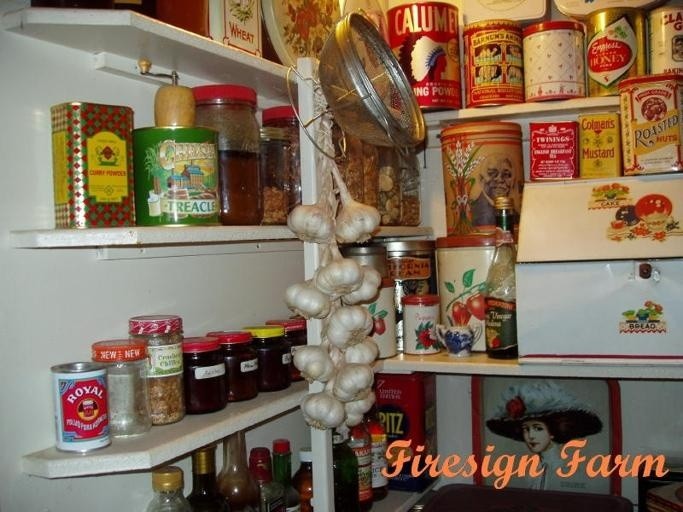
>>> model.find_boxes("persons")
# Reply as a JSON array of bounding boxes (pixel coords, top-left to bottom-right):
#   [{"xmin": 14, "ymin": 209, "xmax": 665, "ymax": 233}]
[
  {"xmin": 517, "ymin": 415, "xmax": 585, "ymax": 495},
  {"xmin": 468, "ymin": 154, "xmax": 520, "ymax": 226}
]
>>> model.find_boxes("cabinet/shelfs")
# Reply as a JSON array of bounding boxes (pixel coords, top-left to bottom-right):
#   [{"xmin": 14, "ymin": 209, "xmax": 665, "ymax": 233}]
[
  {"xmin": 382, "ymin": 95, "xmax": 683, "ymax": 381},
  {"xmin": 0, "ymin": 4, "xmax": 451, "ymax": 512}
]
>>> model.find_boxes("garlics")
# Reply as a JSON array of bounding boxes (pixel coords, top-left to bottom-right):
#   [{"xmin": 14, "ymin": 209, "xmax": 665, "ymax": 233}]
[{"xmin": 286, "ymin": 162, "xmax": 382, "ymax": 429}]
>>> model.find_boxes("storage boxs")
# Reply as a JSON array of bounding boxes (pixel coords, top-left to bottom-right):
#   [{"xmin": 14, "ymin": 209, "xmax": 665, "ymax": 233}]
[{"xmin": 511, "ymin": 172, "xmax": 682, "ymax": 369}]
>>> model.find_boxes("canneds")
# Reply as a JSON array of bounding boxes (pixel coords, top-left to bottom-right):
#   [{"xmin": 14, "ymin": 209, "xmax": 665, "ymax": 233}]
[
  {"xmin": 50, "ymin": 360, "xmax": 113, "ymax": 453},
  {"xmin": 183, "ymin": 319, "xmax": 307, "ymax": 414}
]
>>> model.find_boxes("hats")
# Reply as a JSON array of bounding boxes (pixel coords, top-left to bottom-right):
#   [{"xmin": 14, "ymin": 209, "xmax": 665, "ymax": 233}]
[{"xmin": 482, "ymin": 383, "xmax": 605, "ymax": 445}]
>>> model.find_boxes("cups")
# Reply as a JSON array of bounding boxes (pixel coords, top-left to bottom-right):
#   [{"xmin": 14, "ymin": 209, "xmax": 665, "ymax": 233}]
[{"xmin": 433, "ymin": 323, "xmax": 483, "ymax": 358}]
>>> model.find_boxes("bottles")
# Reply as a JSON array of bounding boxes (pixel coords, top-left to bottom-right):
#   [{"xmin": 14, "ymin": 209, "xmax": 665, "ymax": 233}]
[
  {"xmin": 93, "ymin": 340, "xmax": 153, "ymax": 437},
  {"xmin": 127, "ymin": 311, "xmax": 183, "ymax": 426},
  {"xmin": 146, "ymin": 406, "xmax": 388, "ymax": 512},
  {"xmin": 192, "ymin": 84, "xmax": 421, "ymax": 227},
  {"xmin": 485, "ymin": 196, "xmax": 517, "ymax": 362}
]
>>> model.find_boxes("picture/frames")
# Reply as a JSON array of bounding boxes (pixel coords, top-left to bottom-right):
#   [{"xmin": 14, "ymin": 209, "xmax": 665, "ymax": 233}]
[{"xmin": 467, "ymin": 373, "xmax": 623, "ymax": 501}]
